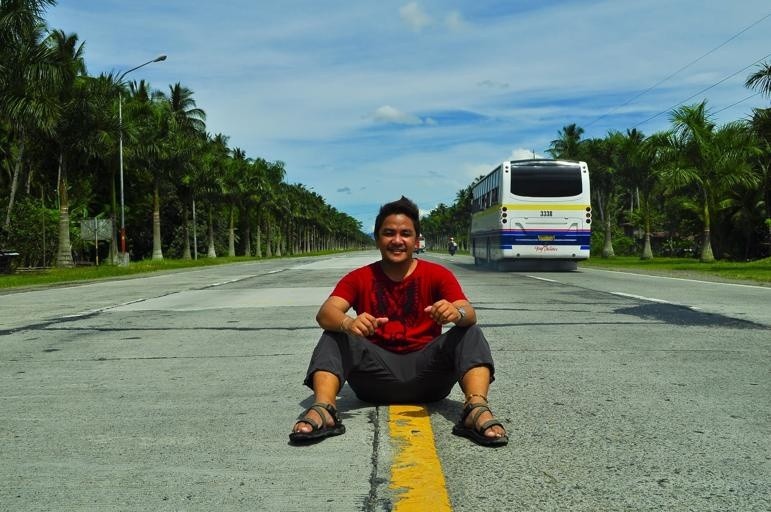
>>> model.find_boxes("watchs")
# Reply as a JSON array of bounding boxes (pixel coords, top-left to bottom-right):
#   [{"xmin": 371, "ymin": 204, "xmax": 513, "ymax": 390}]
[{"xmin": 455, "ymin": 303, "xmax": 466, "ymax": 324}]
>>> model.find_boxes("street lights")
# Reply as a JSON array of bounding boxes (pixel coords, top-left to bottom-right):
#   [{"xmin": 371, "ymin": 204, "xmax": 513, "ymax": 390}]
[{"xmin": 115, "ymin": 54, "xmax": 167, "ymax": 270}]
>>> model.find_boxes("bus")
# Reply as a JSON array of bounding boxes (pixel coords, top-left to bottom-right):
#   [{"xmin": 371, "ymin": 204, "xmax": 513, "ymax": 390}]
[{"xmin": 470, "ymin": 159, "xmax": 592, "ymax": 272}]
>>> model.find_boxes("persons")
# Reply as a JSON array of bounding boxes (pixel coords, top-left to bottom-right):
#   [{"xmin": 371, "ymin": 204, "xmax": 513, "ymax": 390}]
[
  {"xmin": 447, "ymin": 236, "xmax": 458, "ymax": 256},
  {"xmin": 289, "ymin": 194, "xmax": 510, "ymax": 446}
]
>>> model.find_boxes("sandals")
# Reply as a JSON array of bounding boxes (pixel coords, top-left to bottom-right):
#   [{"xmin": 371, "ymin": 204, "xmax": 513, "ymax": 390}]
[
  {"xmin": 453, "ymin": 403, "xmax": 508, "ymax": 445},
  {"xmin": 289, "ymin": 403, "xmax": 345, "ymax": 445}
]
